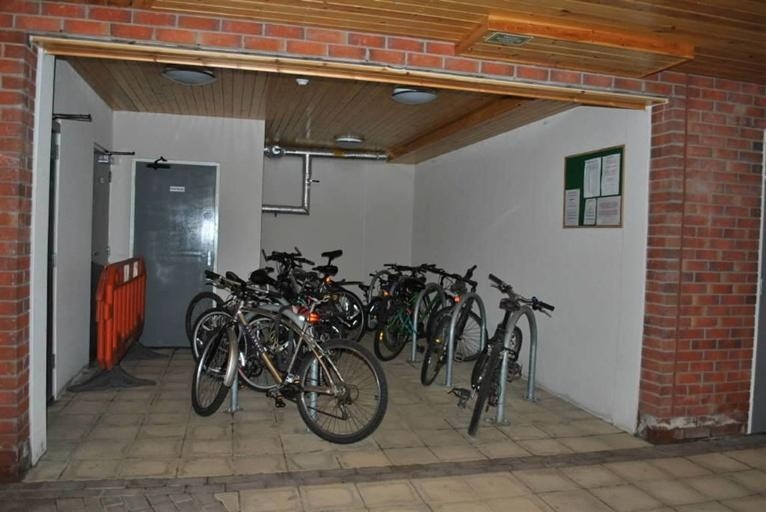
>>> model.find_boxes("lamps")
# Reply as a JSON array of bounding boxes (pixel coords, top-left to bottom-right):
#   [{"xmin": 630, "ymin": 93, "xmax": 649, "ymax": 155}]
[{"xmin": 391, "ymin": 84, "xmax": 440, "ymax": 105}]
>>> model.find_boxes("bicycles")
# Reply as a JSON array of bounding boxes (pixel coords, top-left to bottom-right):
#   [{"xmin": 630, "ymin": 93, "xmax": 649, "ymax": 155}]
[{"xmin": 366, "ymin": 260, "xmax": 554, "ymax": 436}]
